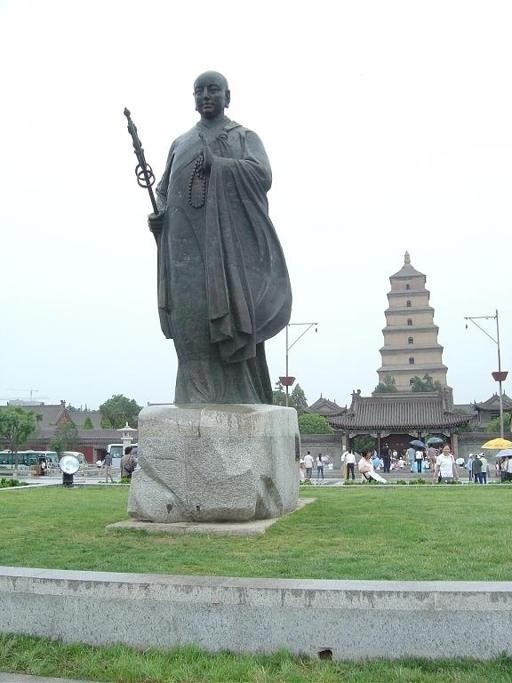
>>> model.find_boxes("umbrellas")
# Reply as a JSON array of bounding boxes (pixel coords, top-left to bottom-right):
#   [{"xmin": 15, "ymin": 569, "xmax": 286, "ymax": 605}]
[{"xmin": 481, "ymin": 438, "xmax": 512, "ymax": 450}]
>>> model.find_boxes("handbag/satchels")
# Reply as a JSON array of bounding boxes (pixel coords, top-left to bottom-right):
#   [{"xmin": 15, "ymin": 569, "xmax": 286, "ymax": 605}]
[{"xmin": 123, "ymin": 462, "xmax": 135, "ymax": 473}]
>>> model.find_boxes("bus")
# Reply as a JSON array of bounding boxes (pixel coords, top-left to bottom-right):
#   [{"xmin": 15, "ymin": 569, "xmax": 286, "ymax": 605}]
[
  {"xmin": 104, "ymin": 443, "xmax": 139, "ymax": 469},
  {"xmin": 0, "ymin": 449, "xmax": 87, "ymax": 477}
]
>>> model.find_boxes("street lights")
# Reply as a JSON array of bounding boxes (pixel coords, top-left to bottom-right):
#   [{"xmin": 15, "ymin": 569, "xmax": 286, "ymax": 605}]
[
  {"xmin": 464, "ymin": 308, "xmax": 509, "ymax": 439},
  {"xmin": 279, "ymin": 320, "xmax": 319, "ymax": 407}
]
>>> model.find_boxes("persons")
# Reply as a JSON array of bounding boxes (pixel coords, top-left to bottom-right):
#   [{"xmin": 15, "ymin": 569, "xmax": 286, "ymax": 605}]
[
  {"xmin": 26, "ymin": 456, "xmax": 46, "ymax": 475},
  {"xmin": 97, "ymin": 445, "xmax": 137, "ymax": 483},
  {"xmin": 122, "ymin": 69, "xmax": 292, "ymax": 402},
  {"xmin": 340, "ymin": 441, "xmax": 512, "ymax": 485},
  {"xmin": 299, "ymin": 450, "xmax": 325, "ymax": 482}
]
